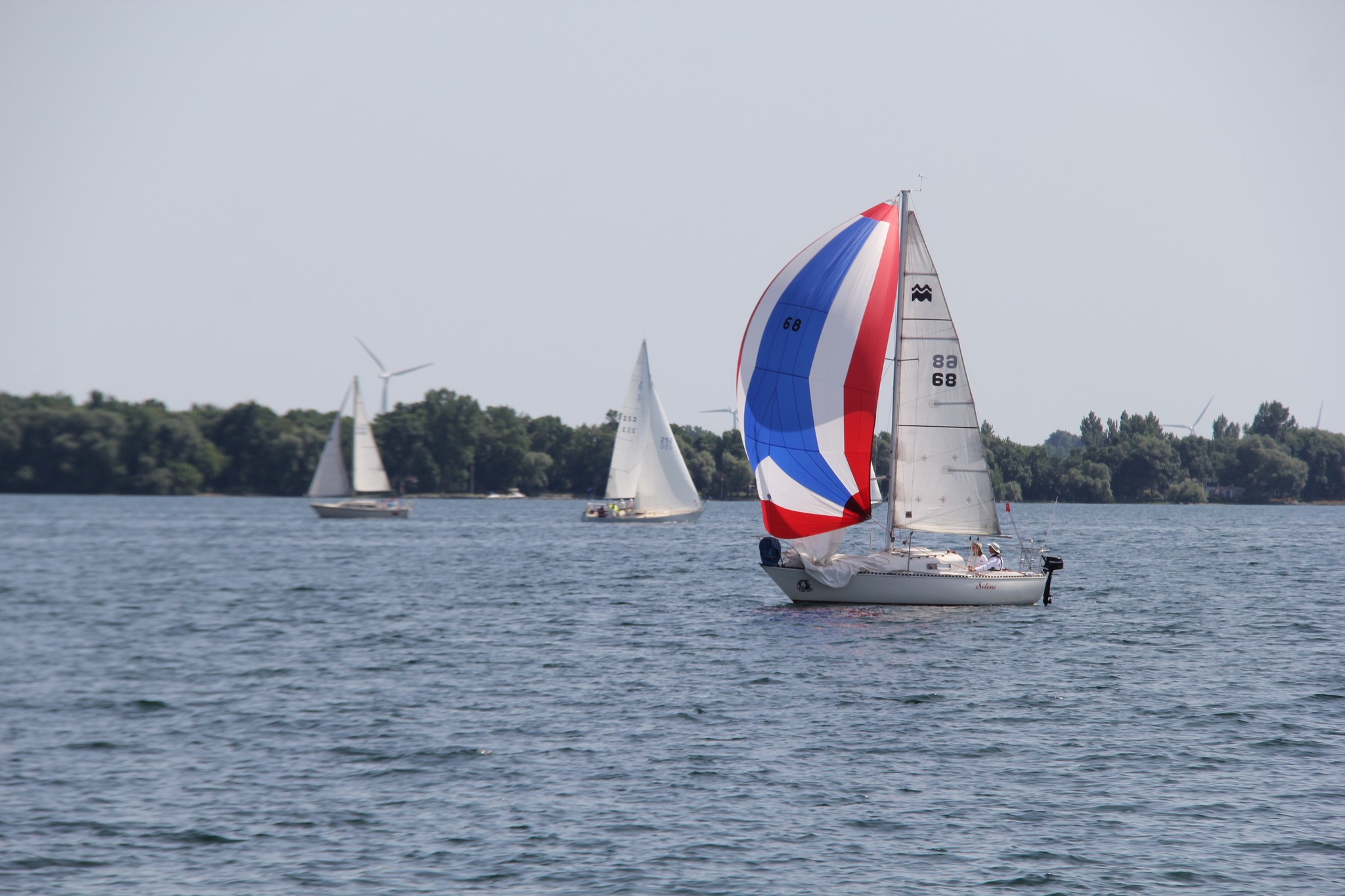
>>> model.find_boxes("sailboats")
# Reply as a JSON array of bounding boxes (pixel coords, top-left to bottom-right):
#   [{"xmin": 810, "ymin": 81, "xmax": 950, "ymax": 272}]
[
  {"xmin": 580, "ymin": 336, "xmax": 707, "ymax": 524},
  {"xmin": 302, "ymin": 374, "xmax": 412, "ymax": 519},
  {"xmin": 732, "ymin": 188, "xmax": 1067, "ymax": 605}
]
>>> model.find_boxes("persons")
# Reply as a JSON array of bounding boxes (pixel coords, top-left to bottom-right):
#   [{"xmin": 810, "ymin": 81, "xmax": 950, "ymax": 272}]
[
  {"xmin": 965, "ymin": 541, "xmax": 988, "ymax": 569},
  {"xmin": 968, "ymin": 542, "xmax": 1003, "ymax": 572},
  {"xmin": 596, "ymin": 497, "xmax": 634, "ymax": 518}
]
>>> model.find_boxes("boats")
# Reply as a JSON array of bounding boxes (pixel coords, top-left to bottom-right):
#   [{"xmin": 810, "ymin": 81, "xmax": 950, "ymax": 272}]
[{"xmin": 485, "ymin": 487, "xmax": 527, "ymax": 500}]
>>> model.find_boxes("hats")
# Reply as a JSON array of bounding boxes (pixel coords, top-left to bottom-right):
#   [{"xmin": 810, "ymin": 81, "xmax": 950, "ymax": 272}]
[
  {"xmin": 972, "ymin": 541, "xmax": 983, "ymax": 557},
  {"xmin": 988, "ymin": 542, "xmax": 1000, "ymax": 553}
]
